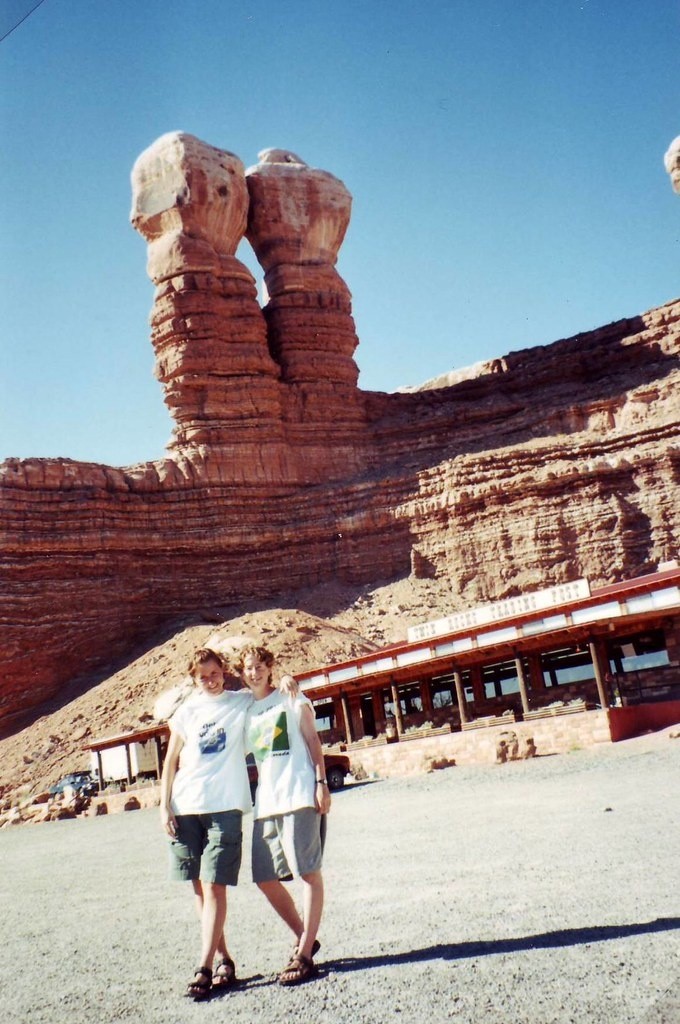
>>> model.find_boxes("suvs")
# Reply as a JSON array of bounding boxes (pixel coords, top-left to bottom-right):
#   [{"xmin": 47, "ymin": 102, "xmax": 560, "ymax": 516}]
[{"xmin": 49, "ymin": 771, "xmax": 98, "ymax": 798}]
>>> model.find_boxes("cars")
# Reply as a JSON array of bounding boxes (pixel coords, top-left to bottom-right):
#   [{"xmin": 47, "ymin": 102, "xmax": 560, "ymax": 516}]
[{"xmin": 246, "ymin": 752, "xmax": 351, "ymax": 806}]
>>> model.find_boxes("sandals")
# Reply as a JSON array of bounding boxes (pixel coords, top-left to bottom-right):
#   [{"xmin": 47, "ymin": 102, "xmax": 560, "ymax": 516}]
[
  {"xmin": 187, "ymin": 967, "xmax": 213, "ymax": 997},
  {"xmin": 211, "ymin": 958, "xmax": 235, "ymax": 991},
  {"xmin": 279, "ymin": 955, "xmax": 315, "ymax": 985},
  {"xmin": 288, "ymin": 939, "xmax": 321, "ymax": 966}
]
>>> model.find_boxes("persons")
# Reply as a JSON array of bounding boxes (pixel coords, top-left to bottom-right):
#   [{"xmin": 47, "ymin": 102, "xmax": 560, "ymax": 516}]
[
  {"xmin": 160, "ymin": 648, "xmax": 298, "ymax": 997},
  {"xmin": 605, "ymin": 672, "xmax": 622, "ymax": 699},
  {"xmin": 234, "ymin": 646, "xmax": 331, "ymax": 983}
]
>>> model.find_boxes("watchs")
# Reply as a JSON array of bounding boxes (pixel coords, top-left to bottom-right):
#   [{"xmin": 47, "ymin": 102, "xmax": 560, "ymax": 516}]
[{"xmin": 316, "ymin": 779, "xmax": 327, "ymax": 784}]
